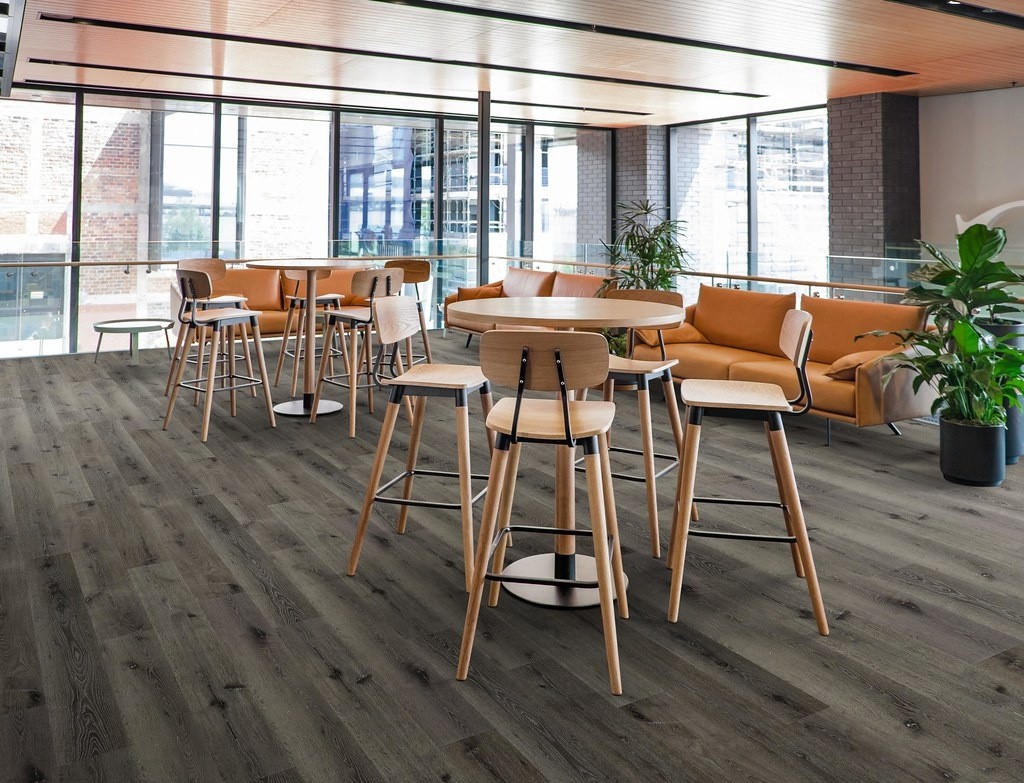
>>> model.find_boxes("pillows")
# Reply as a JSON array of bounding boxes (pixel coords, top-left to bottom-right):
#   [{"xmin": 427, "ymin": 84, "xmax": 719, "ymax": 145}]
[
  {"xmin": 456, "ymin": 285, "xmax": 505, "ymax": 304},
  {"xmin": 823, "ymin": 350, "xmax": 895, "ymax": 381},
  {"xmin": 628, "ymin": 322, "xmax": 709, "ymax": 347}
]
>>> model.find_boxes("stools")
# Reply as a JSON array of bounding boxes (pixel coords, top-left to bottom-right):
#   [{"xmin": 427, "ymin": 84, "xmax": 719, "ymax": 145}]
[{"xmin": 164, "ymin": 258, "xmax": 829, "ymax": 696}]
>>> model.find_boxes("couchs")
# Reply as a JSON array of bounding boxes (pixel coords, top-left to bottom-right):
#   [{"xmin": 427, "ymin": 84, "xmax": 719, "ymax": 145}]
[
  {"xmin": 168, "ymin": 268, "xmax": 394, "ymax": 345},
  {"xmin": 626, "ymin": 282, "xmax": 951, "ymax": 446},
  {"xmin": 444, "ymin": 265, "xmax": 616, "ymax": 347}
]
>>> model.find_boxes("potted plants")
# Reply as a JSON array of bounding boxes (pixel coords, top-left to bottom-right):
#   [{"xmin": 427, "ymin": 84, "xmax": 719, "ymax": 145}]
[
  {"xmin": 854, "ymin": 223, "xmax": 1024, "ymax": 488},
  {"xmin": 592, "ymin": 199, "xmax": 695, "ymax": 392}
]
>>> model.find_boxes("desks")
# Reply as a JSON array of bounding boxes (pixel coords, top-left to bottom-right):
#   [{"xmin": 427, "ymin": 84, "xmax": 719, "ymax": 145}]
[
  {"xmin": 94, "ymin": 318, "xmax": 175, "ymax": 368},
  {"xmin": 245, "ymin": 260, "xmax": 376, "ymax": 418},
  {"xmin": 447, "ymin": 297, "xmax": 687, "ymax": 611}
]
